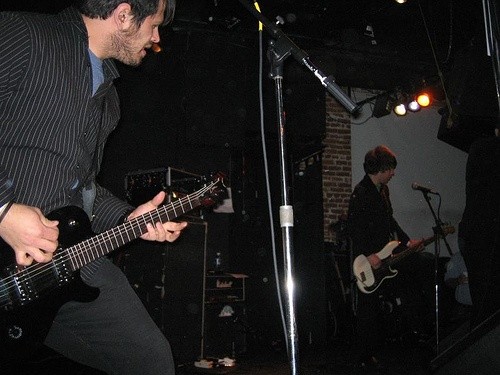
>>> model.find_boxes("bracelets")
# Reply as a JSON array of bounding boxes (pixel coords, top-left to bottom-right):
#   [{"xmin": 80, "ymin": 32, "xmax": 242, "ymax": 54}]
[{"xmin": 0, "ymin": 198, "xmax": 16, "ymax": 223}]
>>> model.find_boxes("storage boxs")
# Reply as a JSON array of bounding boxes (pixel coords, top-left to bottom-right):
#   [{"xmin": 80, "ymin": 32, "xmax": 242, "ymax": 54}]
[{"xmin": 124, "ymin": 166, "xmax": 205, "ymax": 222}]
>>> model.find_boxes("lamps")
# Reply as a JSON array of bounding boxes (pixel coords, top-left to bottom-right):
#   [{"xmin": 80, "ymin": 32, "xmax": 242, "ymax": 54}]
[
  {"xmin": 408, "ymin": 95, "xmax": 422, "ymax": 112},
  {"xmin": 418, "ymin": 92, "xmax": 431, "ymax": 106},
  {"xmin": 394, "ymin": 100, "xmax": 408, "ymax": 116}
]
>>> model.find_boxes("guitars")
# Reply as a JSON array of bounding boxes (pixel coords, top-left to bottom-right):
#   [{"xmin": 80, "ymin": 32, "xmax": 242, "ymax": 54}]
[
  {"xmin": 0, "ymin": 173, "xmax": 230, "ymax": 369},
  {"xmin": 353, "ymin": 221, "xmax": 456, "ymax": 295}
]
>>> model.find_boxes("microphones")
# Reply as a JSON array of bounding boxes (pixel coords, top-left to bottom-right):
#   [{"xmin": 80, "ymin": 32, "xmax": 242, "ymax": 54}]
[{"xmin": 412, "ymin": 182, "xmax": 441, "ymax": 196}]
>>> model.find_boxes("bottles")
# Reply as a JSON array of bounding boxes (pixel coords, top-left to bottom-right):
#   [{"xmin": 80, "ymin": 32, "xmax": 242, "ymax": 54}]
[{"xmin": 213, "ymin": 252, "xmax": 224, "ymax": 288}]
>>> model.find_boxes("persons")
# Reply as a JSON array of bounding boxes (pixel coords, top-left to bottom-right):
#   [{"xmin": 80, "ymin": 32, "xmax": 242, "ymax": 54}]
[
  {"xmin": 455, "ymin": 102, "xmax": 499, "ymax": 323},
  {"xmin": 0, "ymin": 0, "xmax": 189, "ymax": 374},
  {"xmin": 345, "ymin": 144, "xmax": 437, "ymax": 334}
]
